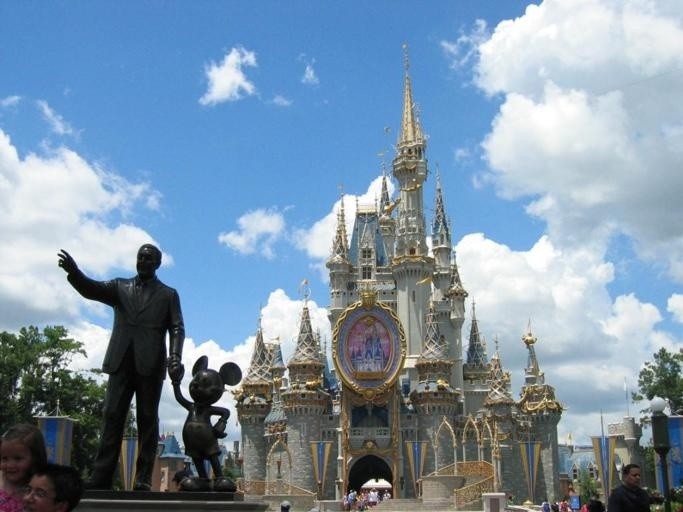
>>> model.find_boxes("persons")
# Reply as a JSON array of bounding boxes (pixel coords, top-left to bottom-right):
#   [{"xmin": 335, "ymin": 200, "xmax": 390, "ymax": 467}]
[
  {"xmin": 0, "ymin": 423, "xmax": 81, "ymax": 512},
  {"xmin": 56, "ymin": 243, "xmax": 185, "ymax": 491},
  {"xmin": 343, "ymin": 487, "xmax": 391, "ymax": 512},
  {"xmin": 542, "ymin": 463, "xmax": 651, "ymax": 512}
]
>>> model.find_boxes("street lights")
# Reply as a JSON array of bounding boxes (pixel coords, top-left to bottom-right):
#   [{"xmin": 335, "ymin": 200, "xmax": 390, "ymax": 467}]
[{"xmin": 649, "ymin": 397, "xmax": 672, "ymax": 512}]
